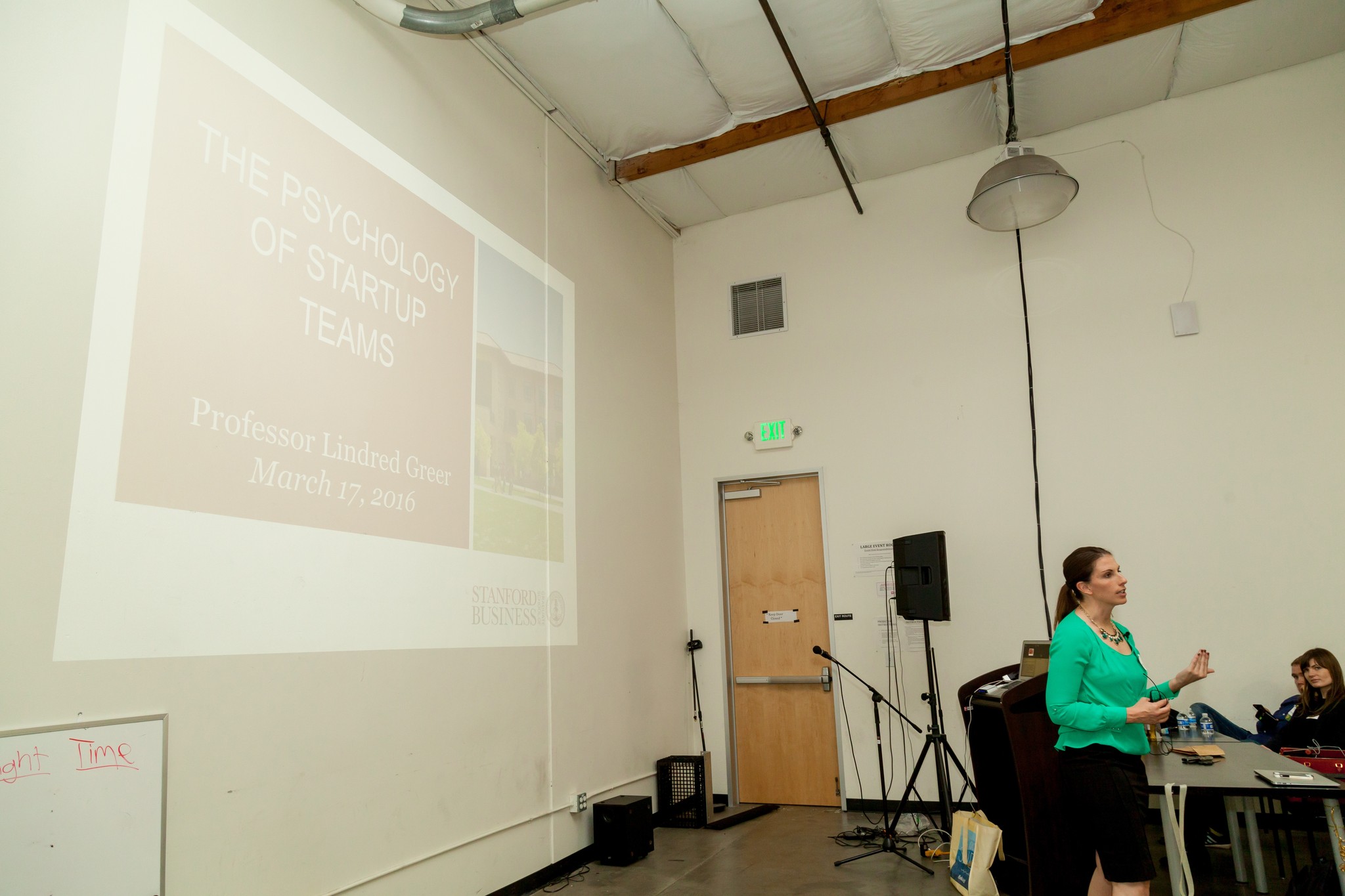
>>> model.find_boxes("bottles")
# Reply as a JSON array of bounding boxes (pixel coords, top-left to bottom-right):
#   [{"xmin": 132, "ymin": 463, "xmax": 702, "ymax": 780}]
[
  {"xmin": 1186, "ymin": 708, "xmax": 1197, "ymax": 728},
  {"xmin": 1200, "ymin": 713, "xmax": 1214, "ymax": 735},
  {"xmin": 1176, "ymin": 709, "xmax": 1190, "ymax": 730},
  {"xmin": 1146, "ymin": 723, "xmax": 1163, "ymax": 739}
]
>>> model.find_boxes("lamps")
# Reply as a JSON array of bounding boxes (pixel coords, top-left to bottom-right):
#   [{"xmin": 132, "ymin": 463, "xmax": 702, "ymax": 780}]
[
  {"xmin": 966, "ymin": 140, "xmax": 1079, "ymax": 232},
  {"xmin": 745, "ymin": 418, "xmax": 802, "ymax": 451}
]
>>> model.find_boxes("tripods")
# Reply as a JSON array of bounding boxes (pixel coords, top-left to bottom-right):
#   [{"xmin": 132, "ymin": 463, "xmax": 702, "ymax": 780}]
[
  {"xmin": 824, "ymin": 651, "xmax": 935, "ymax": 876},
  {"xmin": 891, "ymin": 620, "xmax": 978, "ymax": 842}
]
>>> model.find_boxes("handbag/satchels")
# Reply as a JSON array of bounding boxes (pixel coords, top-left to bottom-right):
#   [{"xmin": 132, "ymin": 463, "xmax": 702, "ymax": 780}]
[{"xmin": 950, "ymin": 809, "xmax": 1005, "ymax": 896}]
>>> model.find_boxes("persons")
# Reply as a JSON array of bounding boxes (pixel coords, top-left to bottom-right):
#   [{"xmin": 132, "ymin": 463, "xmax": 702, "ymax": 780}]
[
  {"xmin": 1158, "ymin": 655, "xmax": 1306, "ymax": 871},
  {"xmin": 1268, "ymin": 648, "xmax": 1345, "ymax": 754},
  {"xmin": 1045, "ymin": 546, "xmax": 1215, "ymax": 896}
]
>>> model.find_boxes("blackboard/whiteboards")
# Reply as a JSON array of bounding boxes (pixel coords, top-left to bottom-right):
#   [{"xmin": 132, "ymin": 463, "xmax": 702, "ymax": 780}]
[{"xmin": 0, "ymin": 714, "xmax": 168, "ymax": 896}]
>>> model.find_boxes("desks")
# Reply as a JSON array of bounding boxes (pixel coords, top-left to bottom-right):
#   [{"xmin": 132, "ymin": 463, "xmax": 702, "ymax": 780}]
[{"xmin": 1141, "ymin": 731, "xmax": 1345, "ymax": 896}]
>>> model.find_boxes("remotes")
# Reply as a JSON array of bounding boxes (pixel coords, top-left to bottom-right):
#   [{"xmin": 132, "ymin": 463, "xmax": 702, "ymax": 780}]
[{"xmin": 1182, "ymin": 755, "xmax": 1213, "ymax": 762}]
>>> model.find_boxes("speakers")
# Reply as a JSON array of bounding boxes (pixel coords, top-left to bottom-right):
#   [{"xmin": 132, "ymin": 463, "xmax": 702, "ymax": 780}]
[
  {"xmin": 593, "ymin": 795, "xmax": 654, "ymax": 866},
  {"xmin": 893, "ymin": 531, "xmax": 952, "ymax": 621}
]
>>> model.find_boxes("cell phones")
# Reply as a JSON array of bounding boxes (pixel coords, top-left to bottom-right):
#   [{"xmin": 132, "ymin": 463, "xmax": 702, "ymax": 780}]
[{"xmin": 1273, "ymin": 772, "xmax": 1314, "ymax": 780}]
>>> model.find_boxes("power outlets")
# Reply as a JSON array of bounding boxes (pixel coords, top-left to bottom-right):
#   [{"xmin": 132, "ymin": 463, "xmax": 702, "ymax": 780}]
[{"xmin": 569, "ymin": 792, "xmax": 587, "ymax": 813}]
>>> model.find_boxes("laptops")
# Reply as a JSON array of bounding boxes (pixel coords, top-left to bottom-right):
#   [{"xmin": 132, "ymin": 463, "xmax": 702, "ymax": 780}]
[
  {"xmin": 1253, "ymin": 769, "xmax": 1340, "ymax": 786},
  {"xmin": 986, "ymin": 640, "xmax": 1052, "ymax": 699}
]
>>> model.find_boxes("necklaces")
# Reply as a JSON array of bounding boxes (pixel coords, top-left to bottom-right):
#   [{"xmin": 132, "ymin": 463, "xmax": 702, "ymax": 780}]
[{"xmin": 1078, "ymin": 604, "xmax": 1123, "ymax": 644}]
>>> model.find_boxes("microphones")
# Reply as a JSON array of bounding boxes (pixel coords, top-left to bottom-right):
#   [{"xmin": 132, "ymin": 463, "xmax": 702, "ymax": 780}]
[
  {"xmin": 1125, "ymin": 631, "xmax": 1130, "ymax": 638},
  {"xmin": 812, "ymin": 645, "xmax": 837, "ymax": 663},
  {"xmin": 1143, "ymin": 673, "xmax": 1161, "ymax": 699}
]
